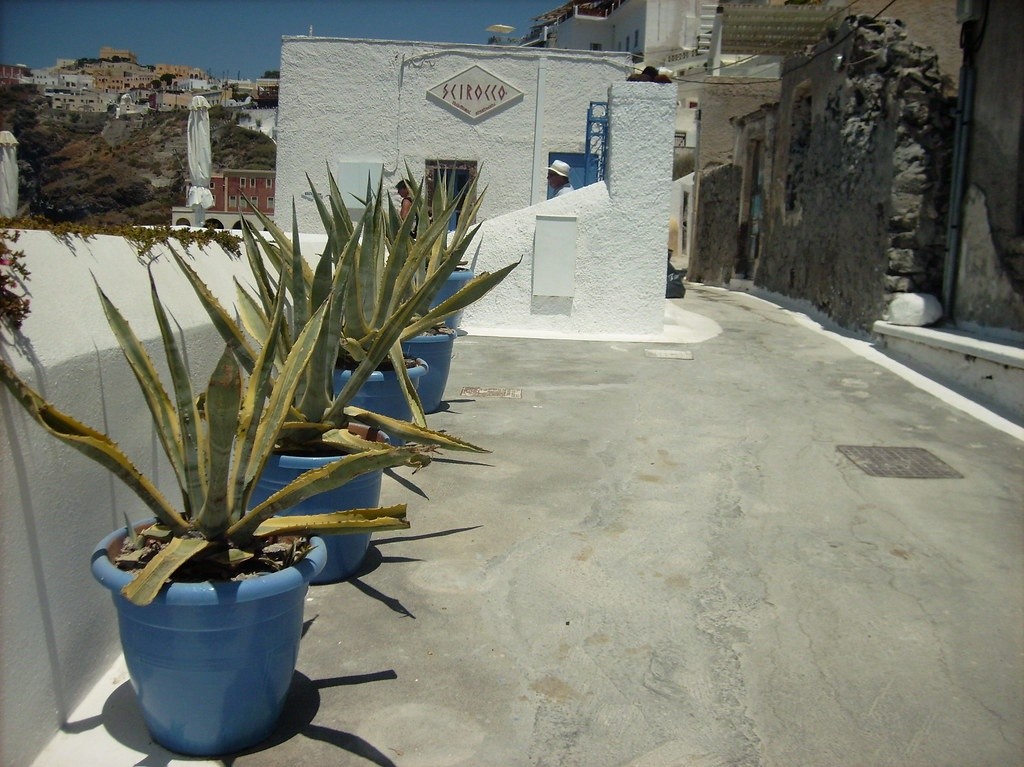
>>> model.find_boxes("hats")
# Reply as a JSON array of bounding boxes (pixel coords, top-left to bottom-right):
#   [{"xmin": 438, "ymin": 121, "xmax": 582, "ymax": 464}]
[{"xmin": 544, "ymin": 159, "xmax": 570, "ymax": 178}]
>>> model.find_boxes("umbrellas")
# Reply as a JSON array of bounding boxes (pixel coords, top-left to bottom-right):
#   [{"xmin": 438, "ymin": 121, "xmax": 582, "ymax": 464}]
[
  {"xmin": 0, "ymin": 131, "xmax": 20, "ymax": 220},
  {"xmin": 484, "ymin": 24, "xmax": 516, "ymax": 37},
  {"xmin": 186, "ymin": 96, "xmax": 215, "ymax": 226}
]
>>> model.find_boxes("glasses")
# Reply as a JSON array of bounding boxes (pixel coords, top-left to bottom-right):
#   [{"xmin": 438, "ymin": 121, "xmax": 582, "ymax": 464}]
[{"xmin": 547, "ymin": 172, "xmax": 559, "ymax": 177}]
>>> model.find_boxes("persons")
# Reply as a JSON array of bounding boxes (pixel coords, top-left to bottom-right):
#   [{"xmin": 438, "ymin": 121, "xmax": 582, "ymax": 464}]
[
  {"xmin": 544, "ymin": 160, "xmax": 574, "ymax": 200},
  {"xmin": 395, "ymin": 179, "xmax": 418, "ymax": 239}
]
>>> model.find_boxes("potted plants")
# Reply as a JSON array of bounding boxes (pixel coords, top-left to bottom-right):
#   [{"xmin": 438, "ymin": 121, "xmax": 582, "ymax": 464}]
[{"xmin": 0, "ymin": 160, "xmax": 523, "ymax": 757}]
